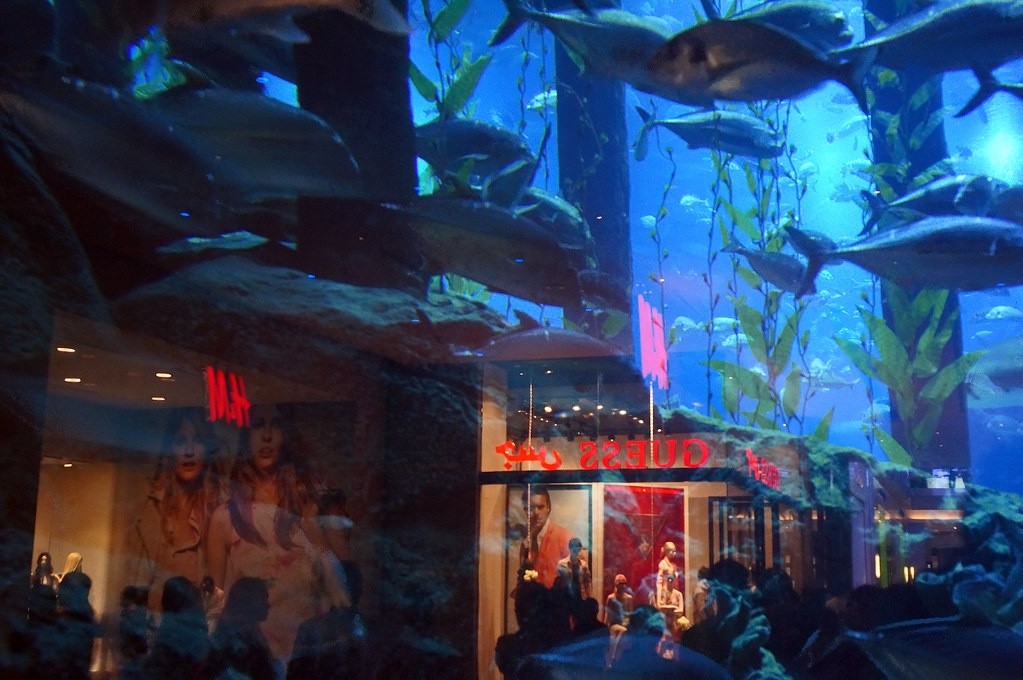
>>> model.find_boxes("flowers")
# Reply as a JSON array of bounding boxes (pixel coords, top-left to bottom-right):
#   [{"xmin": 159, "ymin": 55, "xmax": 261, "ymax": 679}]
[{"xmin": 523, "ymin": 570, "xmax": 539, "ymax": 582}]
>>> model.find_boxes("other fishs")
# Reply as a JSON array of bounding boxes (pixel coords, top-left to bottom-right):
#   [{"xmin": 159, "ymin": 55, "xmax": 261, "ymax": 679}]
[
  {"xmin": 1, "ymin": 0, "xmax": 1023, "ymax": 361},
  {"xmin": 536, "ymin": 633, "xmax": 734, "ymax": 680},
  {"xmin": 799, "ymin": 602, "xmax": 1023, "ymax": 680}
]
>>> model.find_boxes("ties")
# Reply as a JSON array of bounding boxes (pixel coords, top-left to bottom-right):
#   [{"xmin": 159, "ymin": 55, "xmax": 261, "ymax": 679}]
[{"xmin": 530, "ymin": 534, "xmax": 539, "ymax": 560}]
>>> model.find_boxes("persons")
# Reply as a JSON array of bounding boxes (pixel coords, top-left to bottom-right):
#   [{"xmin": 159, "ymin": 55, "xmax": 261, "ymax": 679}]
[
  {"xmin": 129, "ymin": 406, "xmax": 347, "ymax": 654},
  {"xmin": 509, "ymin": 488, "xmax": 585, "ymax": 599},
  {"xmin": 27, "ymin": 572, "xmax": 105, "ymax": 680},
  {"xmin": 119, "ymin": 561, "xmax": 460, "ymax": 680},
  {"xmin": 496, "ymin": 538, "xmax": 942, "ymax": 680},
  {"xmin": 32, "ymin": 552, "xmax": 84, "ymax": 588}
]
